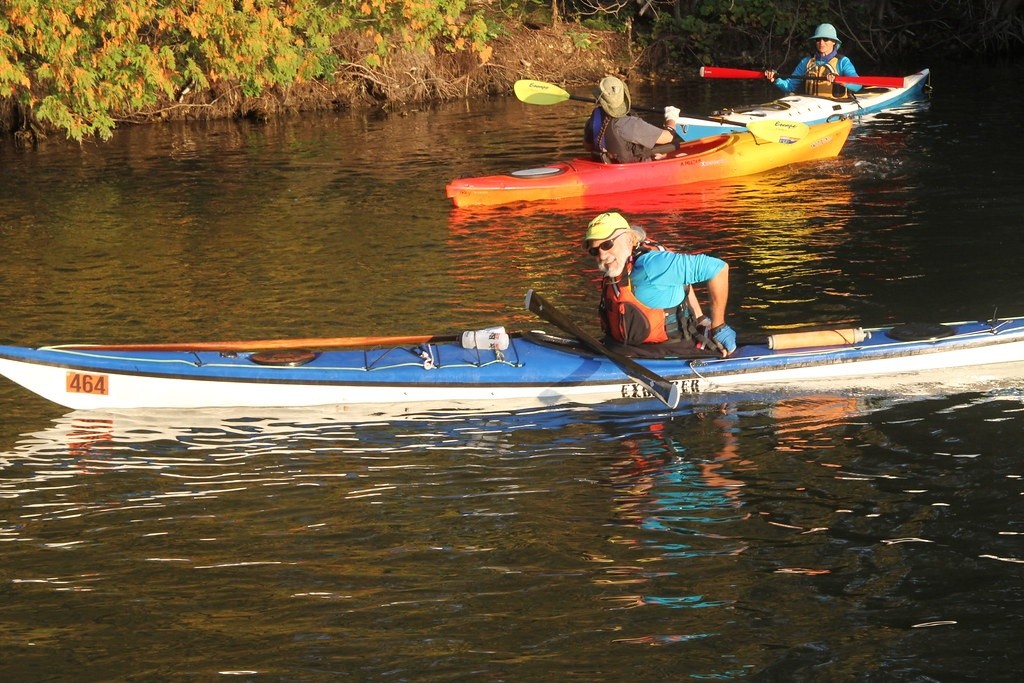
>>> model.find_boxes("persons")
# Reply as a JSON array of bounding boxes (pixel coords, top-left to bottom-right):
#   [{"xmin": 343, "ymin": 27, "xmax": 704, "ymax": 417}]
[
  {"xmin": 581, "ymin": 77, "xmax": 681, "ymax": 167},
  {"xmin": 583, "ymin": 209, "xmax": 737, "ymax": 357},
  {"xmin": 764, "ymin": 21, "xmax": 863, "ymax": 102}
]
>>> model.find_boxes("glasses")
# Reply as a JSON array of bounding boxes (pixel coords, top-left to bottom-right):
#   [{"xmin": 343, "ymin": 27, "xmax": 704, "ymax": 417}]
[
  {"xmin": 589, "ymin": 231, "xmax": 627, "ymax": 256},
  {"xmin": 816, "ymin": 38, "xmax": 829, "ymax": 42}
]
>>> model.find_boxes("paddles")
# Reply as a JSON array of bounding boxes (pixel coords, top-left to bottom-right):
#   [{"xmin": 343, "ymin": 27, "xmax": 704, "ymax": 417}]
[
  {"xmin": 513, "ymin": 75, "xmax": 812, "ymax": 146},
  {"xmin": 522, "ymin": 284, "xmax": 686, "ymax": 410},
  {"xmin": 698, "ymin": 64, "xmax": 918, "ymax": 92},
  {"xmin": 32, "ymin": 318, "xmax": 540, "ymax": 359}
]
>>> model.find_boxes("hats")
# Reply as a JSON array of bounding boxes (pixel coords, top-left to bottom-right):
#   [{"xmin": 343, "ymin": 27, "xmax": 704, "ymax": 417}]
[
  {"xmin": 581, "ymin": 212, "xmax": 630, "ymax": 251},
  {"xmin": 809, "ymin": 23, "xmax": 842, "ymax": 49},
  {"xmin": 592, "ymin": 75, "xmax": 631, "ymax": 118}
]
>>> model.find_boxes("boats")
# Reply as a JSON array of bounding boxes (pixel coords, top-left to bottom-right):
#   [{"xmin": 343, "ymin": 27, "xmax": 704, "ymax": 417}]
[
  {"xmin": 444, "ymin": 115, "xmax": 853, "ymax": 208},
  {"xmin": 0, "ymin": 316, "xmax": 1024, "ymax": 429},
  {"xmin": 672, "ymin": 66, "xmax": 932, "ymax": 143}
]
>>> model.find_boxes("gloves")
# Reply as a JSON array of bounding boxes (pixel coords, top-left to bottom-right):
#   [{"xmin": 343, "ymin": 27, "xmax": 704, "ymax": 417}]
[{"xmin": 663, "ymin": 106, "xmax": 681, "ymax": 123}]
